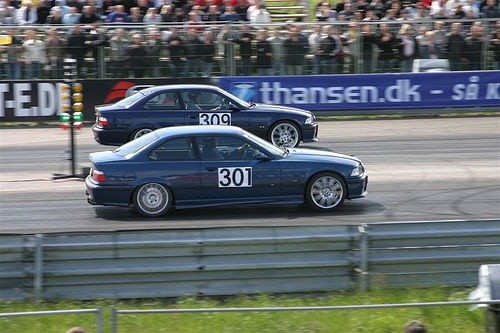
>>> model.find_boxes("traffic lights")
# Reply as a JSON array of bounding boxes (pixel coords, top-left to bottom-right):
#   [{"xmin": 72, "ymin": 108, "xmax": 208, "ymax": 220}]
[{"xmin": 57, "ymin": 82, "xmax": 84, "ymax": 131}]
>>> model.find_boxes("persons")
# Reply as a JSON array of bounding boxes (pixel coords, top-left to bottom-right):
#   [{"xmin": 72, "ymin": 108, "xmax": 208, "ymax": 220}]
[
  {"xmin": 202, "ymin": 139, "xmax": 248, "ymax": 161},
  {"xmin": 182, "ymin": 91, "xmax": 221, "ymax": 111},
  {"xmin": 0, "ymin": 0, "xmax": 499, "ymax": 80}
]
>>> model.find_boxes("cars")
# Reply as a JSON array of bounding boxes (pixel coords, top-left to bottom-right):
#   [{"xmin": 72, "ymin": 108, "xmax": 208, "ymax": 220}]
[
  {"xmin": 92, "ymin": 83, "xmax": 318, "ymax": 152},
  {"xmin": 84, "ymin": 124, "xmax": 368, "ymax": 220}
]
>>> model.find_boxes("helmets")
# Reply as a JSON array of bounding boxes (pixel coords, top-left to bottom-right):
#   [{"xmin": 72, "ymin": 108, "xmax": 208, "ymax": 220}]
[
  {"xmin": 203, "ymin": 135, "xmax": 218, "ymax": 148},
  {"xmin": 187, "ymin": 92, "xmax": 203, "ymax": 104}
]
